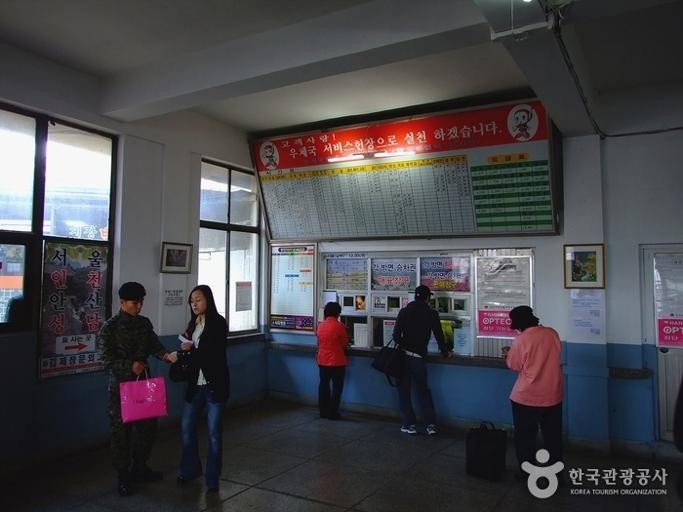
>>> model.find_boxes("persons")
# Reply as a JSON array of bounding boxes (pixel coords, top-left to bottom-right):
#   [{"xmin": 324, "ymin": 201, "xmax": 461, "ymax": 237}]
[
  {"xmin": 315, "ymin": 301, "xmax": 348, "ymax": 421},
  {"xmin": 355, "ymin": 296, "xmax": 365, "ymax": 309},
  {"xmin": 392, "ymin": 285, "xmax": 451, "ymax": 437},
  {"xmin": 166, "ymin": 251, "xmax": 177, "ymax": 266},
  {"xmin": 161, "ymin": 285, "xmax": 229, "ymax": 495},
  {"xmin": 175, "ymin": 249, "xmax": 186, "ymax": 265},
  {"xmin": 95, "ymin": 282, "xmax": 178, "ymax": 497},
  {"xmin": 501, "ymin": 305, "xmax": 563, "ymax": 482}
]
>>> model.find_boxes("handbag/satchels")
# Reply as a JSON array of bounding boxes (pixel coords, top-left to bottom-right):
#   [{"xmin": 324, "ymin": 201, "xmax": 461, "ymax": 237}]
[
  {"xmin": 118, "ymin": 377, "xmax": 169, "ymax": 424},
  {"xmin": 371, "ymin": 347, "xmax": 404, "ymax": 377},
  {"xmin": 465, "ymin": 422, "xmax": 506, "ymax": 478},
  {"xmin": 168, "ymin": 348, "xmax": 201, "ymax": 374}
]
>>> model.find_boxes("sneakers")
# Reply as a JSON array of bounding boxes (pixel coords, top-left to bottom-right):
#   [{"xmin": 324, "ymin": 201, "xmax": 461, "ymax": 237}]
[
  {"xmin": 400, "ymin": 425, "xmax": 417, "ymax": 434},
  {"xmin": 425, "ymin": 424, "xmax": 439, "ymax": 435}
]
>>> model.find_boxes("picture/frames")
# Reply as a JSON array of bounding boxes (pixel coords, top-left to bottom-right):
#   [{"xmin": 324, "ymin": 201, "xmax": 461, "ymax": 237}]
[
  {"xmin": 562, "ymin": 242, "xmax": 606, "ymax": 289},
  {"xmin": 158, "ymin": 240, "xmax": 193, "ymax": 274}
]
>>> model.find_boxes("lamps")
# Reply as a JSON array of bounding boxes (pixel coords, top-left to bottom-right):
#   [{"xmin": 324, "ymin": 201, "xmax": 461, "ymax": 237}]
[{"xmin": 325, "ymin": 149, "xmax": 415, "ymax": 165}]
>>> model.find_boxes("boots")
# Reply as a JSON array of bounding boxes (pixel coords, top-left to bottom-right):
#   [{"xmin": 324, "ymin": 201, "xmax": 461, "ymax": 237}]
[
  {"xmin": 116, "ymin": 469, "xmax": 131, "ymax": 495},
  {"xmin": 132, "ymin": 458, "xmax": 163, "ymax": 481}
]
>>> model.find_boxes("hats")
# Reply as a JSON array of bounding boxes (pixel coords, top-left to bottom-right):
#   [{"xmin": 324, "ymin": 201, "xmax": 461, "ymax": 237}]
[
  {"xmin": 118, "ymin": 282, "xmax": 146, "ymax": 301},
  {"xmin": 509, "ymin": 305, "xmax": 533, "ymax": 319},
  {"xmin": 414, "ymin": 286, "xmax": 435, "ymax": 296}
]
